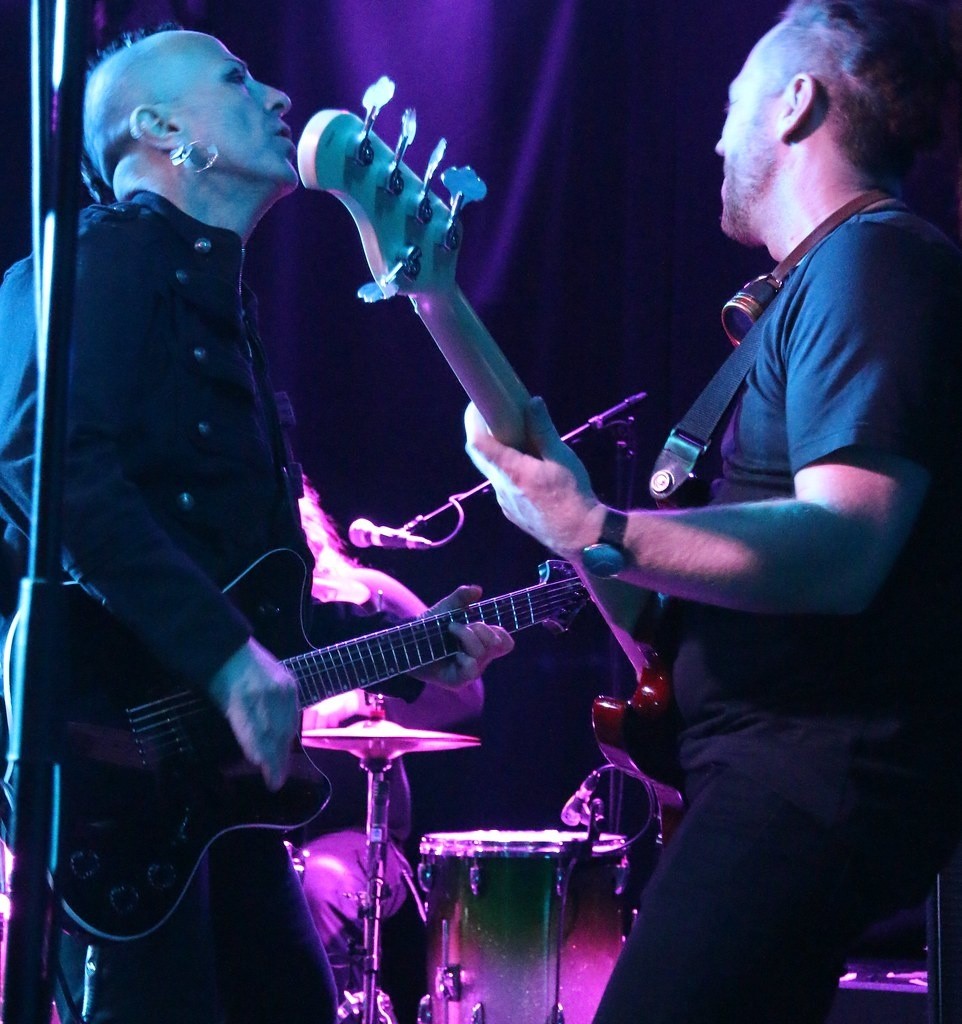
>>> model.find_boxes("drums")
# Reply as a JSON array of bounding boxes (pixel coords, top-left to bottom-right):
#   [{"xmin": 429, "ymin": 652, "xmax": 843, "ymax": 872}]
[{"xmin": 419, "ymin": 826, "xmax": 623, "ymax": 1023}]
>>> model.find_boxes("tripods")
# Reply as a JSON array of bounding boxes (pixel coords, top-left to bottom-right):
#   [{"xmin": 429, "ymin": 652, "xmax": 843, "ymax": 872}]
[{"xmin": 336, "ymin": 773, "xmax": 397, "ymax": 1024}]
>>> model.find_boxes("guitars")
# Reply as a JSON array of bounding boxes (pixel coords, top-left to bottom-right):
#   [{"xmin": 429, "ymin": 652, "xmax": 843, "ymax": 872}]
[
  {"xmin": 294, "ymin": 72, "xmax": 689, "ymax": 810},
  {"xmin": 2, "ymin": 543, "xmax": 600, "ymax": 946}
]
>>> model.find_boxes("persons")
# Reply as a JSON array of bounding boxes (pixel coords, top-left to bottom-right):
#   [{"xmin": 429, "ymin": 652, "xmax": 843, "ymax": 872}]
[{"xmin": 3, "ymin": 0, "xmax": 962, "ymax": 1023}]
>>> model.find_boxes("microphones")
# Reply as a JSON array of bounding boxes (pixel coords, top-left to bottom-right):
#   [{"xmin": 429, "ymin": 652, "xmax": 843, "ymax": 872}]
[
  {"xmin": 368, "ymin": 779, "xmax": 391, "ymax": 879},
  {"xmin": 347, "ymin": 517, "xmax": 430, "ymax": 550},
  {"xmin": 562, "ymin": 774, "xmax": 601, "ymax": 826}
]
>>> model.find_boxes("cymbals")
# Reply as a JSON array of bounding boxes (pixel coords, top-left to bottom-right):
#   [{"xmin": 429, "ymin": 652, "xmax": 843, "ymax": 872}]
[{"xmin": 294, "ymin": 716, "xmax": 483, "ymax": 768}]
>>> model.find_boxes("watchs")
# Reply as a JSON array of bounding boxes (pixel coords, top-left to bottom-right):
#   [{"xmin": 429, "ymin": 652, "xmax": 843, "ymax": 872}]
[{"xmin": 582, "ymin": 507, "xmax": 628, "ymax": 579}]
